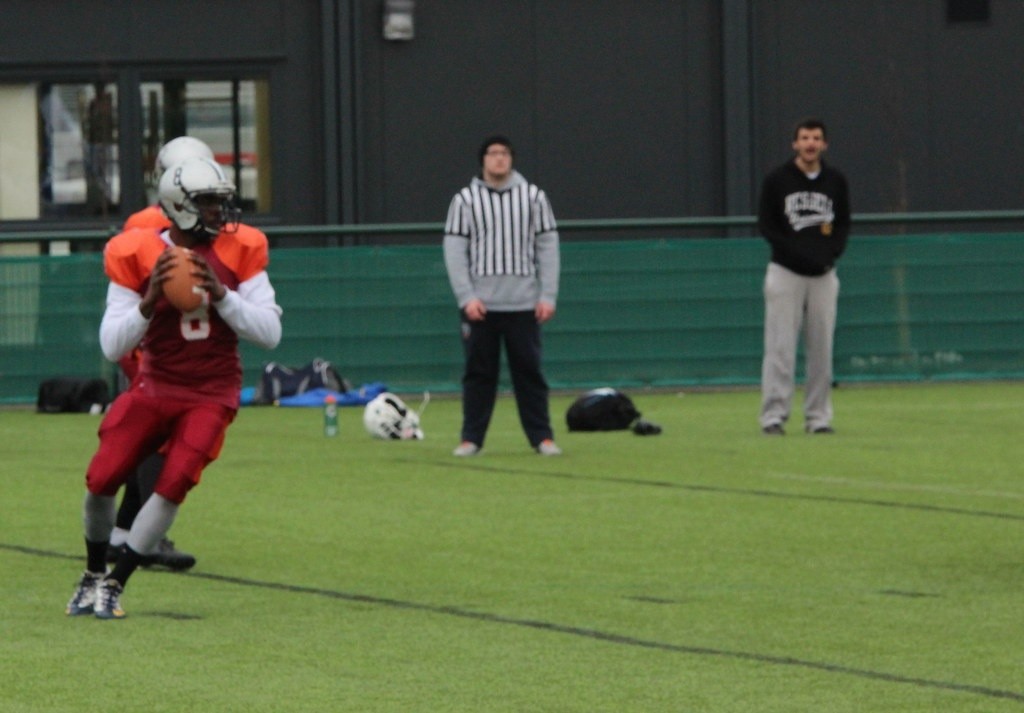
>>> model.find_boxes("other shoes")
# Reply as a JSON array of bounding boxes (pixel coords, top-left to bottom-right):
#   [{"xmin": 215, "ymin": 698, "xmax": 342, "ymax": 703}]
[
  {"xmin": 763, "ymin": 423, "xmax": 784, "ymax": 435},
  {"xmin": 537, "ymin": 438, "xmax": 561, "ymax": 455},
  {"xmin": 454, "ymin": 444, "xmax": 477, "ymax": 457},
  {"xmin": 105, "ymin": 537, "xmax": 176, "ymax": 562},
  {"xmin": 119, "ymin": 543, "xmax": 195, "ymax": 573}
]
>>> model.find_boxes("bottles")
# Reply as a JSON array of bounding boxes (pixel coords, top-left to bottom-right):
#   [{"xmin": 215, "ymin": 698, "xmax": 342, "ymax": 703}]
[{"xmin": 325, "ymin": 397, "xmax": 337, "ymax": 435}]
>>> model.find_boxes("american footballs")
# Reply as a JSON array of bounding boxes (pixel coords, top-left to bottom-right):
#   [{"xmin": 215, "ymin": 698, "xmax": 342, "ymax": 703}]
[{"xmin": 160, "ymin": 247, "xmax": 204, "ymax": 312}]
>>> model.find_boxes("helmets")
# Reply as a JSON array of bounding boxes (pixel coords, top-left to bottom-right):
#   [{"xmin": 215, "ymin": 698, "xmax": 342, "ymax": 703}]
[
  {"xmin": 159, "ymin": 154, "xmax": 237, "ymax": 231},
  {"xmin": 154, "ymin": 136, "xmax": 215, "ymax": 184}
]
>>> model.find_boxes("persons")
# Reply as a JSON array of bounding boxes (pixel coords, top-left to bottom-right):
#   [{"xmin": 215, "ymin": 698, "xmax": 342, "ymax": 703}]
[
  {"xmin": 104, "ymin": 136, "xmax": 215, "ymax": 573},
  {"xmin": 63, "ymin": 155, "xmax": 284, "ymax": 619},
  {"xmin": 443, "ymin": 137, "xmax": 566, "ymax": 457},
  {"xmin": 756, "ymin": 119, "xmax": 854, "ymax": 435}
]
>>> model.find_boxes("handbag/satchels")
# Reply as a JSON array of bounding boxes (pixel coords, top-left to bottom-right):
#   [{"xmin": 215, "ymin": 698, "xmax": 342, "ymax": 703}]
[
  {"xmin": 263, "ymin": 358, "xmax": 350, "ymax": 400},
  {"xmin": 38, "ymin": 379, "xmax": 108, "ymax": 413}
]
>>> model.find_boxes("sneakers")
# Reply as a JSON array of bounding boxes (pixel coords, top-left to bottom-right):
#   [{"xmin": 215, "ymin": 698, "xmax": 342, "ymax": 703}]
[
  {"xmin": 65, "ymin": 566, "xmax": 111, "ymax": 616},
  {"xmin": 93, "ymin": 577, "xmax": 126, "ymax": 619}
]
